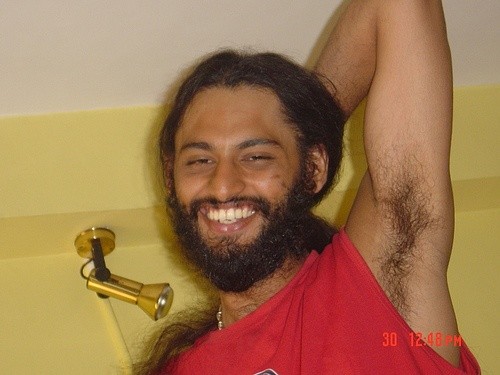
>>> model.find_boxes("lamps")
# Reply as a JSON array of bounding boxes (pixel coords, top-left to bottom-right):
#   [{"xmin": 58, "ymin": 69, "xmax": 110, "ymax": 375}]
[{"xmin": 74, "ymin": 226, "xmax": 175, "ymax": 323}]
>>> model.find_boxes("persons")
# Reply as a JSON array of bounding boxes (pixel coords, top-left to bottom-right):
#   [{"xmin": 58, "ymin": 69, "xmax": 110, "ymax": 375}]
[{"xmin": 131, "ymin": 0, "xmax": 483, "ymax": 374}]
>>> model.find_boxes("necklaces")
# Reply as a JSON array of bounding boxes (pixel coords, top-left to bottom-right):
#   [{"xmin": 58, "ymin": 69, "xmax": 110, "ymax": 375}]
[{"xmin": 215, "ymin": 303, "xmax": 225, "ymax": 335}]
